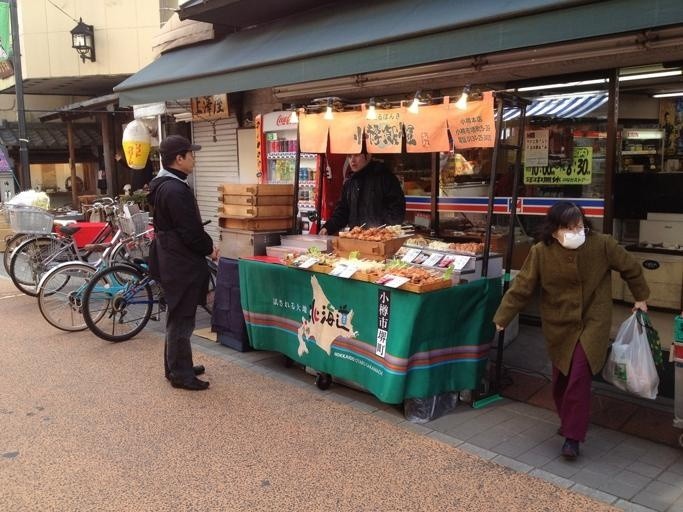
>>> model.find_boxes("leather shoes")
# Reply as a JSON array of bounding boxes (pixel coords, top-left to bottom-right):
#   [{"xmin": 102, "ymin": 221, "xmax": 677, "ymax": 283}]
[
  {"xmin": 561, "ymin": 438, "xmax": 580, "ymax": 461},
  {"xmin": 166, "ymin": 365, "xmax": 205, "ymax": 381},
  {"xmin": 171, "ymin": 378, "xmax": 209, "ymax": 390}
]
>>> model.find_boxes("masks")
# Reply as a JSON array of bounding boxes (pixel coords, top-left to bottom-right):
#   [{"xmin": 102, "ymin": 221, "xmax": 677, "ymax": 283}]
[{"xmin": 562, "ymin": 231, "xmax": 585, "ymax": 249}]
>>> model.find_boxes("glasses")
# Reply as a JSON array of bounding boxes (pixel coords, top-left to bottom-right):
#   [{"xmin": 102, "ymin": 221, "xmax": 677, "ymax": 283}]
[{"xmin": 560, "ymin": 227, "xmax": 590, "ymax": 239}]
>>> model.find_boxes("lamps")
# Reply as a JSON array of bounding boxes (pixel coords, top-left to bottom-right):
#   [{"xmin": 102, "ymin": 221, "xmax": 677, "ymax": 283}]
[
  {"xmin": 406, "ymin": 91, "xmax": 422, "ymax": 114},
  {"xmin": 70, "ymin": 16, "xmax": 96, "ymax": 64},
  {"xmin": 365, "ymin": 98, "xmax": 377, "ymax": 121},
  {"xmin": 453, "ymin": 85, "xmax": 471, "ymax": 111},
  {"xmin": 324, "ymin": 98, "xmax": 334, "ymax": 120},
  {"xmin": 289, "ymin": 105, "xmax": 299, "ymax": 124}
]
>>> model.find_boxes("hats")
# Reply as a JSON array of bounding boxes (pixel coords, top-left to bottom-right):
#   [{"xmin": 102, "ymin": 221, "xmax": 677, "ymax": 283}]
[{"xmin": 160, "ymin": 135, "xmax": 202, "ymax": 155}]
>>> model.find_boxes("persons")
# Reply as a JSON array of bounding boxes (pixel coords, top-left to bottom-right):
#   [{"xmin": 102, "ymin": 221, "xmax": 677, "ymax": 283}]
[
  {"xmin": 317, "ymin": 141, "xmax": 406, "ymax": 235},
  {"xmin": 493, "ymin": 204, "xmax": 651, "ymax": 460},
  {"xmin": 146, "ymin": 136, "xmax": 219, "ymax": 390}
]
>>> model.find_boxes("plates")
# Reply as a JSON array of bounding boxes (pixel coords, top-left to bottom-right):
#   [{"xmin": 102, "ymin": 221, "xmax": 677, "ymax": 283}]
[
  {"xmin": 284, "ymin": 258, "xmax": 327, "ymax": 274},
  {"xmin": 369, "ymin": 269, "xmax": 452, "ymax": 293},
  {"xmin": 327, "ymin": 266, "xmax": 370, "ymax": 283},
  {"xmin": 332, "ymin": 236, "xmax": 415, "ymax": 256}
]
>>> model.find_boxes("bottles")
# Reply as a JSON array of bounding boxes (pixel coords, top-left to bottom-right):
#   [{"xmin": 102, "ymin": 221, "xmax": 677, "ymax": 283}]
[
  {"xmin": 269, "ymin": 140, "xmax": 297, "ymax": 153},
  {"xmin": 299, "ymin": 167, "xmax": 315, "ymax": 181},
  {"xmin": 298, "ymin": 184, "xmax": 313, "ymax": 201}
]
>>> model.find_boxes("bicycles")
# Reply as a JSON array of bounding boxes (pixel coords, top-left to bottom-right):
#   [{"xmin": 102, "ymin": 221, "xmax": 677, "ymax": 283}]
[
  {"xmin": 10, "ymin": 195, "xmax": 150, "ymax": 298},
  {"xmin": 3, "ymin": 203, "xmax": 126, "ymax": 287},
  {"xmin": 37, "ymin": 223, "xmax": 156, "ymax": 331},
  {"xmin": 82, "ymin": 219, "xmax": 221, "ymax": 342}
]
